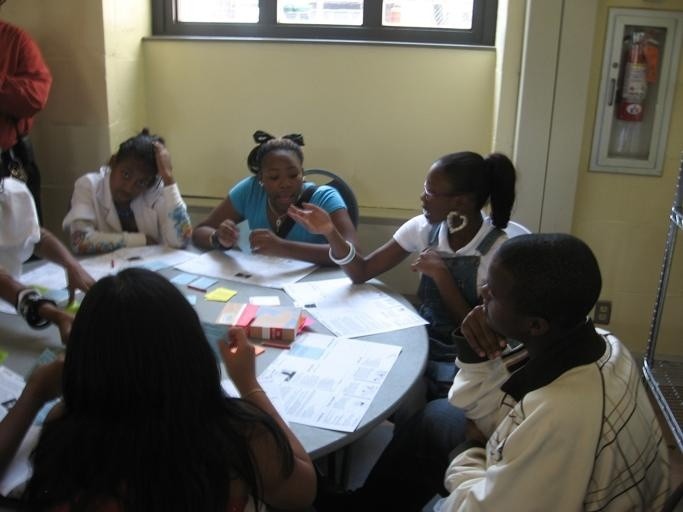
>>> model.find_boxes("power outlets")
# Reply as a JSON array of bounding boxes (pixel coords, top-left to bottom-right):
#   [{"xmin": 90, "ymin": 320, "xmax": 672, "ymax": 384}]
[{"xmin": 593, "ymin": 301, "xmax": 609, "ymax": 324}]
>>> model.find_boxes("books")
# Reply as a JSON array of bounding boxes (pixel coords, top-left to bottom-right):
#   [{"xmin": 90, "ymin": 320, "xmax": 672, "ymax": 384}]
[{"xmin": 216, "ymin": 300, "xmax": 302, "ymax": 342}]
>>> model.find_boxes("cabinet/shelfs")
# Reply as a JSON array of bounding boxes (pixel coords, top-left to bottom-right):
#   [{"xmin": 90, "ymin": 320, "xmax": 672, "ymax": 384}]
[{"xmin": 641, "ymin": 157, "xmax": 683, "ymax": 454}]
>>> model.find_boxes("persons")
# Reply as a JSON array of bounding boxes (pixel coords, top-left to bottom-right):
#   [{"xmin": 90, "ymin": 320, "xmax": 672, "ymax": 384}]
[
  {"xmin": 287, "ymin": 151, "xmax": 532, "ymax": 401},
  {"xmin": 312, "ymin": 233, "xmax": 682, "ymax": 512},
  {"xmin": 0, "ymin": 0, "xmax": 53, "ymax": 264},
  {"xmin": 0, "ymin": 158, "xmax": 95, "ymax": 345},
  {"xmin": 0, "ymin": 268, "xmax": 318, "ymax": 511},
  {"xmin": 192, "ymin": 130, "xmax": 361, "ymax": 267},
  {"xmin": 61, "ymin": 128, "xmax": 192, "ymax": 256}
]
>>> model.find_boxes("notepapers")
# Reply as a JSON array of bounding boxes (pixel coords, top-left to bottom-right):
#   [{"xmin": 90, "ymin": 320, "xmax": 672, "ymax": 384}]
[
  {"xmin": 190, "ymin": 277, "xmax": 216, "ymax": 288},
  {"xmin": 248, "ymin": 296, "xmax": 280, "ymax": 305},
  {"xmin": 205, "ymin": 288, "xmax": 237, "ymax": 302},
  {"xmin": 232, "ymin": 342, "xmax": 265, "ymax": 356},
  {"xmin": 171, "ymin": 274, "xmax": 198, "ymax": 285}
]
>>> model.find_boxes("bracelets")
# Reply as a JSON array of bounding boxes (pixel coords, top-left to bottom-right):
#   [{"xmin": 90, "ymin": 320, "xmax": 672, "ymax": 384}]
[
  {"xmin": 241, "ymin": 388, "xmax": 264, "ymax": 401},
  {"xmin": 328, "ymin": 240, "xmax": 355, "ymax": 265},
  {"xmin": 14, "ymin": 287, "xmax": 56, "ymax": 330},
  {"xmin": 208, "ymin": 230, "xmax": 232, "ymax": 250}
]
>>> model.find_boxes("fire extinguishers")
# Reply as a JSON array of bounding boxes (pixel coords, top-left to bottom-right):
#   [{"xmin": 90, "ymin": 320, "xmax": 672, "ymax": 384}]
[{"xmin": 617, "ymin": 31, "xmax": 660, "ymax": 121}]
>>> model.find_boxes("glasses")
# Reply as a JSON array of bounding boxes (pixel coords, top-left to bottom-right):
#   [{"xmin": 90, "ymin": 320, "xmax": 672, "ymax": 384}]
[{"xmin": 424, "ymin": 181, "xmax": 456, "ymax": 201}]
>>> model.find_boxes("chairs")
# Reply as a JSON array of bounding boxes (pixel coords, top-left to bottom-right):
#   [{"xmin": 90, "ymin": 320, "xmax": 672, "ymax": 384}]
[
  {"xmin": 301, "ymin": 167, "xmax": 359, "ymax": 233},
  {"xmin": 484, "ymin": 213, "xmax": 532, "ymax": 240}
]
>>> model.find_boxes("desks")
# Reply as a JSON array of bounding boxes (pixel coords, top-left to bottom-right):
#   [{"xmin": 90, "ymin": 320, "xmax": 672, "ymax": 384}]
[{"xmin": 0, "ymin": 234, "xmax": 430, "ymax": 511}]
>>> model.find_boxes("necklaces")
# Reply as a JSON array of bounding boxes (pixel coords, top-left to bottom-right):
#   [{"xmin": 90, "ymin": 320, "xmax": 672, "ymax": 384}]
[
  {"xmin": 267, "ymin": 194, "xmax": 301, "ymax": 233},
  {"xmin": 117, "ymin": 207, "xmax": 133, "ymax": 219}
]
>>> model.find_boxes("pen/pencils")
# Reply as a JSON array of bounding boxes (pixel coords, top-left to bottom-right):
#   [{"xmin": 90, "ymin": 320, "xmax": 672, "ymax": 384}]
[
  {"xmin": 188, "ymin": 286, "xmax": 205, "ymax": 292},
  {"xmin": 227, "ymin": 316, "xmax": 257, "ymax": 350},
  {"xmin": 111, "ymin": 250, "xmax": 115, "ymax": 269},
  {"xmin": 260, "ymin": 342, "xmax": 289, "ymax": 349}
]
